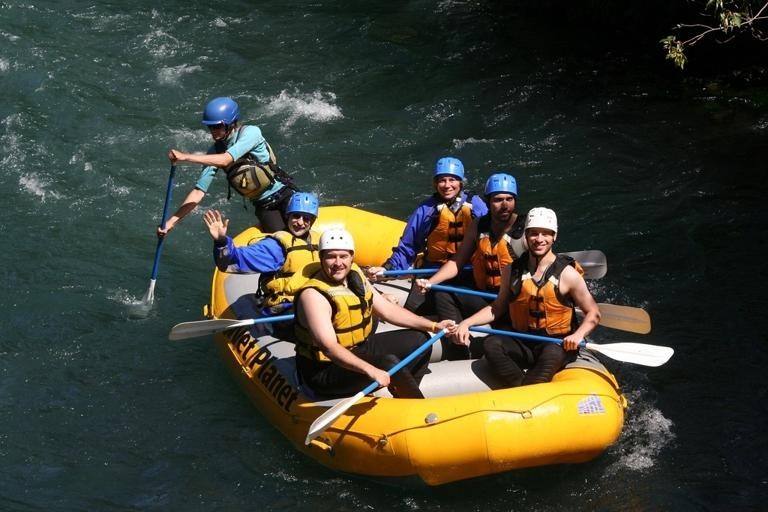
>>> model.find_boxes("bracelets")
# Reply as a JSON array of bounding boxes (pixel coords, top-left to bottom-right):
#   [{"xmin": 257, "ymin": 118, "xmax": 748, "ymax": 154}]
[{"xmin": 431, "ymin": 321, "xmax": 436, "ymax": 334}]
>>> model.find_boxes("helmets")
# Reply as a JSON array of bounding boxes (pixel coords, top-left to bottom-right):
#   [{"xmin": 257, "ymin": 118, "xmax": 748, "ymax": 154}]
[
  {"xmin": 285, "ymin": 191, "xmax": 319, "ymax": 219},
  {"xmin": 483, "ymin": 172, "xmax": 518, "ymax": 198},
  {"xmin": 201, "ymin": 96, "xmax": 241, "ymax": 127},
  {"xmin": 524, "ymin": 206, "xmax": 559, "ymax": 242},
  {"xmin": 432, "ymin": 156, "xmax": 465, "ymax": 181},
  {"xmin": 318, "ymin": 228, "xmax": 355, "ymax": 256}
]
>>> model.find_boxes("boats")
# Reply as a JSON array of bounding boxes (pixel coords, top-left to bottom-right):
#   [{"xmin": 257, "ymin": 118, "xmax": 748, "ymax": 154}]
[{"xmin": 203, "ymin": 205, "xmax": 627, "ymax": 477}]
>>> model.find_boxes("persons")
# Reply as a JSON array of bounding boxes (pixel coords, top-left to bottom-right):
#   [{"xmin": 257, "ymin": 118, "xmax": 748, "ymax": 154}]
[
  {"xmin": 294, "ymin": 227, "xmax": 458, "ymax": 397},
  {"xmin": 203, "ymin": 193, "xmax": 324, "ymax": 316},
  {"xmin": 452, "ymin": 207, "xmax": 602, "ymax": 388},
  {"xmin": 157, "ymin": 97, "xmax": 294, "ymax": 231},
  {"xmin": 414, "ymin": 174, "xmax": 531, "ymax": 325},
  {"xmin": 366, "ymin": 158, "xmax": 488, "ymax": 310}
]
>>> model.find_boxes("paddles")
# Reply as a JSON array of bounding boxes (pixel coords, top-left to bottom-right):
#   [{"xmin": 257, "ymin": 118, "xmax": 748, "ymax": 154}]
[
  {"xmin": 167, "ymin": 313, "xmax": 294, "ymax": 341},
  {"xmin": 304, "ymin": 327, "xmax": 449, "ymax": 446},
  {"xmin": 469, "ymin": 326, "xmax": 674, "ymax": 367},
  {"xmin": 141, "ymin": 159, "xmax": 176, "ymax": 307},
  {"xmin": 423, "ymin": 283, "xmax": 651, "ymax": 334},
  {"xmin": 377, "ymin": 250, "xmax": 609, "ymax": 280}
]
